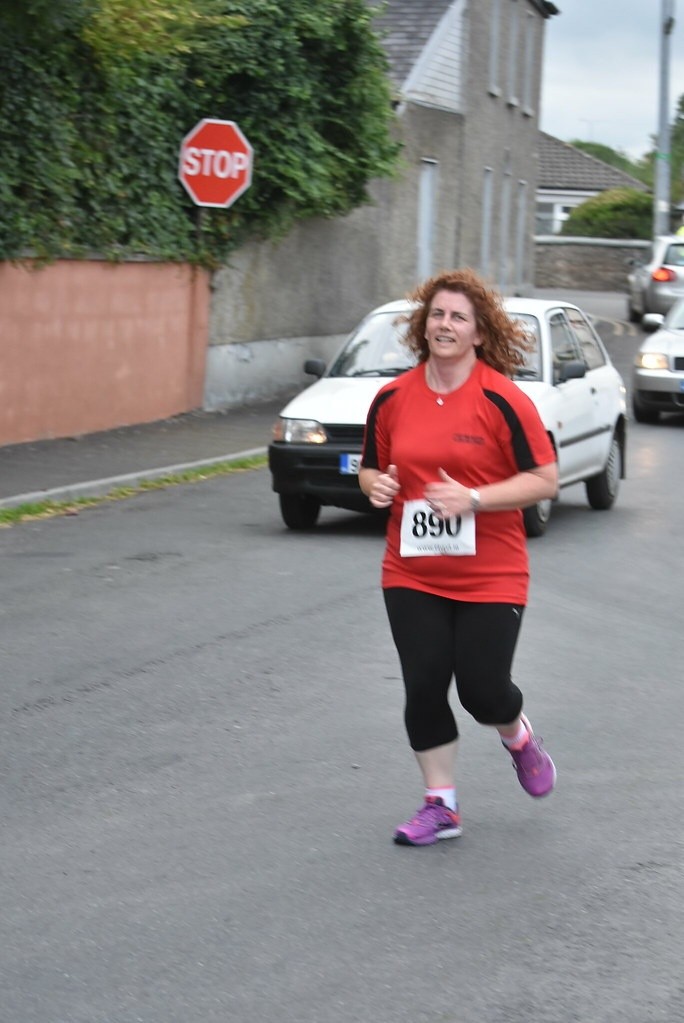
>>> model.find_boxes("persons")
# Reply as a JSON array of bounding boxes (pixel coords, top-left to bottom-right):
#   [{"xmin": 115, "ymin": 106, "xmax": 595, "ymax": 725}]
[{"xmin": 357, "ymin": 267, "xmax": 558, "ymax": 846}]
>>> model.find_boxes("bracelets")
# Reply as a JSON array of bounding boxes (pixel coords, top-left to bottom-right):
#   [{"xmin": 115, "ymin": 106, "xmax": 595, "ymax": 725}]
[{"xmin": 470, "ymin": 487, "xmax": 480, "ymax": 512}]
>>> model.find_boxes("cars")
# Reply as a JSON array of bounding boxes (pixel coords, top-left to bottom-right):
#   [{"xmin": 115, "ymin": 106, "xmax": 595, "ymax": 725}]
[
  {"xmin": 268, "ymin": 296, "xmax": 633, "ymax": 538},
  {"xmin": 632, "ymin": 297, "xmax": 684, "ymax": 425},
  {"xmin": 623, "ymin": 236, "xmax": 684, "ymax": 322}
]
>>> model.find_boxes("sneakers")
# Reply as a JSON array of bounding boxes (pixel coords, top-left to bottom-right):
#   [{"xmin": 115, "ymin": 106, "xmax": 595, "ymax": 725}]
[
  {"xmin": 499, "ymin": 711, "xmax": 555, "ymax": 798},
  {"xmin": 393, "ymin": 802, "xmax": 463, "ymax": 846}
]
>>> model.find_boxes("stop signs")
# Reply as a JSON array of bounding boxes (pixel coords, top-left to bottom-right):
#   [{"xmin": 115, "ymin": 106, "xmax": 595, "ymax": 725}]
[{"xmin": 176, "ymin": 117, "xmax": 254, "ymax": 207}]
